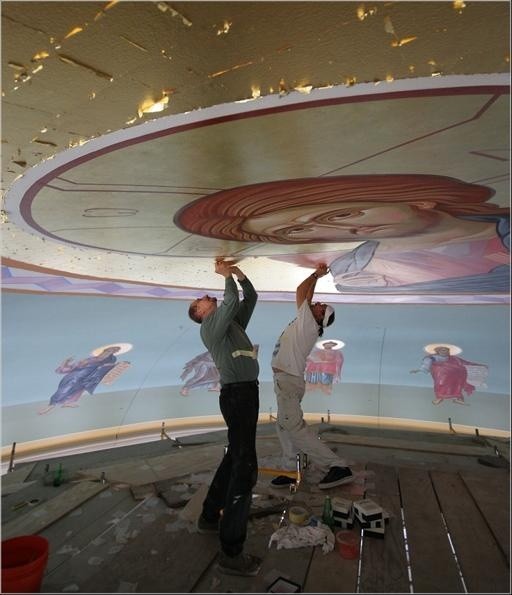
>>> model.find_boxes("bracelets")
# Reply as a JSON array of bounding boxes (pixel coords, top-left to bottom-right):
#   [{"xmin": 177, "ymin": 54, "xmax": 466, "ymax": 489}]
[{"xmin": 311, "ymin": 273, "xmax": 319, "ymax": 279}]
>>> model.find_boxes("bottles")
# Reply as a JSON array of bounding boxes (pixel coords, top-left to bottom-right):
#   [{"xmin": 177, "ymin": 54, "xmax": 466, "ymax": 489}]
[
  {"xmin": 323, "ymin": 495, "xmax": 334, "ymax": 533},
  {"xmin": 295, "ymin": 453, "xmax": 307, "ymax": 486}
]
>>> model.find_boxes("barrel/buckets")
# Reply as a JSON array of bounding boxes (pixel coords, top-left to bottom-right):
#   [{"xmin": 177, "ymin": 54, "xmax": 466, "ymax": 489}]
[
  {"xmin": 336, "ymin": 531, "xmax": 359, "ymax": 559},
  {"xmin": 1, "ymin": 535, "xmax": 49, "ymax": 595}
]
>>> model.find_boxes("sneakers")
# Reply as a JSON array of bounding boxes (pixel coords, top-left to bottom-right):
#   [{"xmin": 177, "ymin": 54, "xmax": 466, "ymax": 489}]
[
  {"xmin": 271, "ymin": 476, "xmax": 297, "ymax": 487},
  {"xmin": 218, "ymin": 553, "xmax": 261, "ymax": 576},
  {"xmin": 197, "ymin": 515, "xmax": 222, "ymax": 533},
  {"xmin": 318, "ymin": 467, "xmax": 352, "ymax": 489}
]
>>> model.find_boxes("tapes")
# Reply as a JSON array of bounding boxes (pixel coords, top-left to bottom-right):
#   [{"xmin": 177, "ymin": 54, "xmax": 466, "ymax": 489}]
[
  {"xmin": 288, "ymin": 506, "xmax": 309, "ymax": 524},
  {"xmin": 28, "ymin": 498, "xmax": 41, "ymax": 506}
]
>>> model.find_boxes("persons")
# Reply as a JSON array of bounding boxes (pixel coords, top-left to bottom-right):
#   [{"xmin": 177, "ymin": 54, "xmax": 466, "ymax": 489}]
[
  {"xmin": 173, "ymin": 175, "xmax": 510, "ymax": 294},
  {"xmin": 180, "ymin": 352, "xmax": 221, "ymax": 396},
  {"xmin": 305, "ymin": 340, "xmax": 345, "ymax": 396},
  {"xmin": 38, "ymin": 342, "xmax": 133, "ymax": 414},
  {"xmin": 411, "ymin": 343, "xmax": 488, "ymax": 405},
  {"xmin": 189, "ymin": 260, "xmax": 259, "ymax": 572},
  {"xmin": 269, "ymin": 262, "xmax": 355, "ymax": 490}
]
